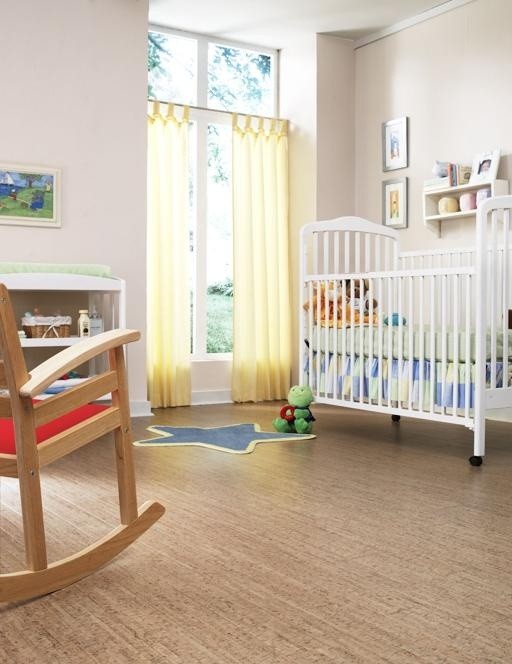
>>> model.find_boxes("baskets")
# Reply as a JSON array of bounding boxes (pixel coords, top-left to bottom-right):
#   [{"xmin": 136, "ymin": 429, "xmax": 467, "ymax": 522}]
[{"xmin": 22, "ymin": 324, "xmax": 71, "ymax": 338}]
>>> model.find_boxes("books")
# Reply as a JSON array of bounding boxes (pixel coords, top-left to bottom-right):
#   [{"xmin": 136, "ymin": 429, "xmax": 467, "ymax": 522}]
[{"xmin": 424, "ymin": 162, "xmax": 472, "ymax": 192}]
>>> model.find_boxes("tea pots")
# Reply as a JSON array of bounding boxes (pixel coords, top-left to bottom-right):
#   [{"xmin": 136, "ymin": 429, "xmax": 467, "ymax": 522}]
[{"xmin": 437, "ymin": 198, "xmax": 459, "ymax": 214}]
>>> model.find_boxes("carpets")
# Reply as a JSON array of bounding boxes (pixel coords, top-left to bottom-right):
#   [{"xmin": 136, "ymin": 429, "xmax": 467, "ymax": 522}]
[{"xmin": 134, "ymin": 423, "xmax": 317, "ymax": 456}]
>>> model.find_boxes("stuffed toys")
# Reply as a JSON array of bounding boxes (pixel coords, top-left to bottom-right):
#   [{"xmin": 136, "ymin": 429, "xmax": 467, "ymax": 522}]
[
  {"xmin": 340, "ymin": 279, "xmax": 378, "ymax": 316},
  {"xmin": 274, "ymin": 385, "xmax": 315, "ymax": 434}
]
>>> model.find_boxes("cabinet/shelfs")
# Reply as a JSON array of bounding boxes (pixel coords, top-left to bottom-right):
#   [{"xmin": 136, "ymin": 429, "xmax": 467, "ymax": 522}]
[
  {"xmin": 0, "ymin": 261, "xmax": 126, "ymax": 402},
  {"xmin": 422, "ymin": 179, "xmax": 509, "ymax": 237}
]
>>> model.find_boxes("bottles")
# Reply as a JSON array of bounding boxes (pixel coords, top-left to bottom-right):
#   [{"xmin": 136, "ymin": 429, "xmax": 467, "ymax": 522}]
[{"xmin": 78, "ymin": 310, "xmax": 102, "ymax": 337}]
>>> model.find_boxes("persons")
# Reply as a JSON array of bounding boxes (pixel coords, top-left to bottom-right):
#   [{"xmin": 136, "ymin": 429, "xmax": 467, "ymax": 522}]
[{"xmin": 479, "ymin": 159, "xmax": 491, "ymax": 179}]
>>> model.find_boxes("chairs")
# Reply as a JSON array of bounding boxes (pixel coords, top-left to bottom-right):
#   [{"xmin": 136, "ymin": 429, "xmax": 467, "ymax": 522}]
[{"xmin": 1, "ymin": 282, "xmax": 165, "ymax": 605}]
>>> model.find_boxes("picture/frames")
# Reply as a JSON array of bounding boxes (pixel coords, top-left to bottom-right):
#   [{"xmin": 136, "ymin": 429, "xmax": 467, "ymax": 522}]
[
  {"xmin": 381, "ymin": 176, "xmax": 408, "ymax": 229},
  {"xmin": 0, "ymin": 164, "xmax": 61, "ymax": 229},
  {"xmin": 467, "ymin": 146, "xmax": 501, "ymax": 183},
  {"xmin": 381, "ymin": 116, "xmax": 409, "ymax": 171}
]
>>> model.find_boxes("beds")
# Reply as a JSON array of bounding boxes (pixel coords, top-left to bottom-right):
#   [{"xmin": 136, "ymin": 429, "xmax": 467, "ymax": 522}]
[{"xmin": 295, "ymin": 194, "xmax": 512, "ymax": 466}]
